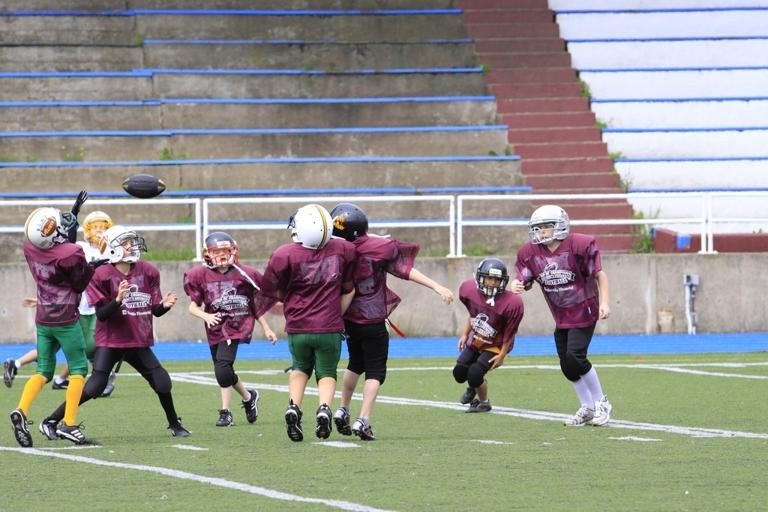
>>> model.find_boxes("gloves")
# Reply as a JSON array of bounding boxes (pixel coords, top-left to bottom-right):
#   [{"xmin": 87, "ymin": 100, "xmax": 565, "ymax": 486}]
[{"xmin": 72, "ymin": 191, "xmax": 87, "ymax": 215}]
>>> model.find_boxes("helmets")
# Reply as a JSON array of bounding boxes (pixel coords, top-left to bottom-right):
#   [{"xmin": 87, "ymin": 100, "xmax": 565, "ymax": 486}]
[
  {"xmin": 529, "ymin": 205, "xmax": 569, "ymax": 244},
  {"xmin": 476, "ymin": 259, "xmax": 509, "ymax": 296},
  {"xmin": 288, "ymin": 204, "xmax": 367, "ymax": 250},
  {"xmin": 203, "ymin": 232, "xmax": 239, "ymax": 269},
  {"xmin": 24, "ymin": 207, "xmax": 147, "ymax": 264}
]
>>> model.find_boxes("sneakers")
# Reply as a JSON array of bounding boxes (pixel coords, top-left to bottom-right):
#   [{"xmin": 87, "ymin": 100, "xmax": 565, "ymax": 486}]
[
  {"xmin": 11, "ymin": 408, "xmax": 85, "ymax": 447},
  {"xmin": 240, "ymin": 390, "xmax": 259, "ymax": 423},
  {"xmin": 101, "ymin": 369, "xmax": 115, "ymax": 396},
  {"xmin": 285, "ymin": 399, "xmax": 375, "ymax": 441},
  {"xmin": 216, "ymin": 410, "xmax": 233, "ymax": 426},
  {"xmin": 168, "ymin": 417, "xmax": 190, "ymax": 436},
  {"xmin": 461, "ymin": 388, "xmax": 491, "ymax": 413},
  {"xmin": 564, "ymin": 394, "xmax": 612, "ymax": 427},
  {"xmin": 4, "ymin": 360, "xmax": 17, "ymax": 387},
  {"xmin": 52, "ymin": 378, "xmax": 68, "ymax": 389}
]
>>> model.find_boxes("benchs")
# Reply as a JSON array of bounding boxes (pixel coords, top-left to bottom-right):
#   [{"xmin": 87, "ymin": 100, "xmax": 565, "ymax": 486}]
[
  {"xmin": 0, "ymin": 0, "xmax": 537, "ymax": 251},
  {"xmin": 549, "ymin": 0, "xmax": 768, "ymax": 249}
]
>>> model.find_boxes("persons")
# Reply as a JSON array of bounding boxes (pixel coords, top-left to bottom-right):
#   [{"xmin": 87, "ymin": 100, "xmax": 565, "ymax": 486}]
[
  {"xmin": 4, "ymin": 211, "xmax": 114, "ymax": 389},
  {"xmin": 86, "ymin": 313, "xmax": 117, "ymax": 397},
  {"xmin": 183, "ymin": 232, "xmax": 277, "ymax": 429},
  {"xmin": 10, "ymin": 190, "xmax": 110, "ymax": 447},
  {"xmin": 453, "ymin": 259, "xmax": 524, "ymax": 413},
  {"xmin": 39, "ymin": 225, "xmax": 191, "ymax": 440},
  {"xmin": 510, "ymin": 205, "xmax": 613, "ymax": 427},
  {"xmin": 260, "ymin": 203, "xmax": 360, "ymax": 441},
  {"xmin": 330, "ymin": 202, "xmax": 453, "ymax": 440}
]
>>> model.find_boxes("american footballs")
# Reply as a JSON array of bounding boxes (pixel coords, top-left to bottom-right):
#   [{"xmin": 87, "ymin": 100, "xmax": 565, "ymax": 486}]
[{"xmin": 122, "ymin": 175, "xmax": 166, "ymax": 198}]
